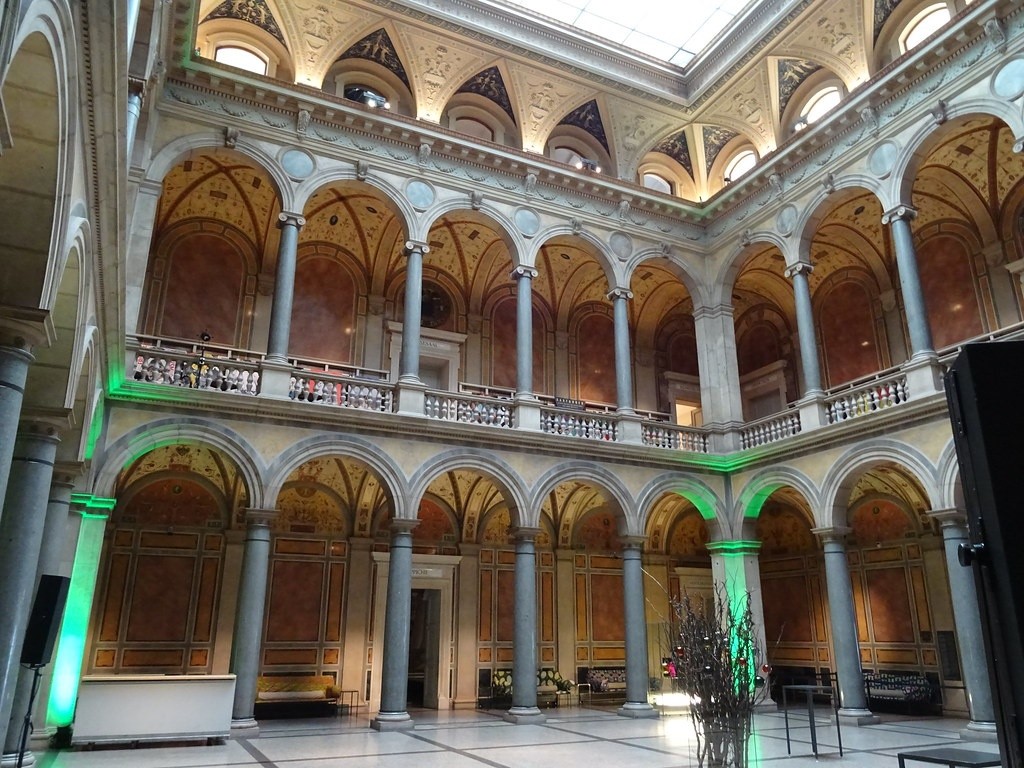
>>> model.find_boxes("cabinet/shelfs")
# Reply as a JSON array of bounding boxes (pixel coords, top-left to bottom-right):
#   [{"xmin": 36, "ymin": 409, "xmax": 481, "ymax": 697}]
[
  {"xmin": 899, "ymin": 748, "xmax": 1001, "ymax": 768},
  {"xmin": 783, "ymin": 685, "xmax": 842, "ymax": 760},
  {"xmin": 494, "ymin": 668, "xmax": 560, "ymax": 708},
  {"xmin": 71, "ymin": 675, "xmax": 237, "ymax": 743}
]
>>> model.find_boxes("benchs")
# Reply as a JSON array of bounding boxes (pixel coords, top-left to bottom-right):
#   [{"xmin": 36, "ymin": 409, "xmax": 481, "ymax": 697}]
[
  {"xmin": 862, "ymin": 672, "xmax": 935, "ymax": 710},
  {"xmin": 577, "ymin": 667, "xmax": 626, "ymax": 704},
  {"xmin": 254, "ymin": 675, "xmax": 340, "ymax": 719}
]
